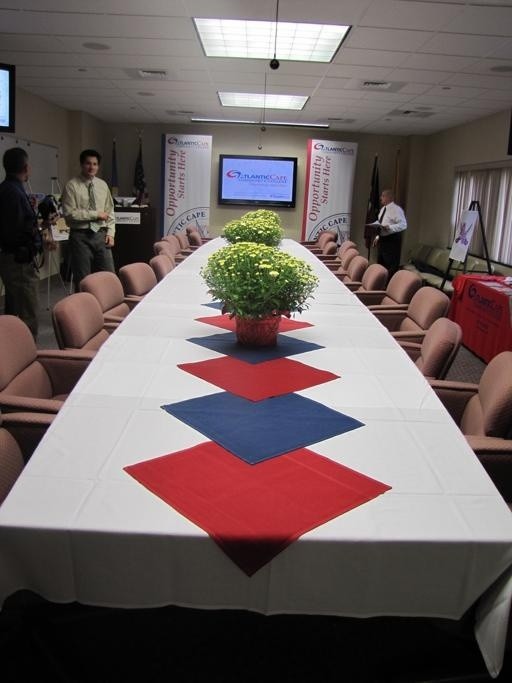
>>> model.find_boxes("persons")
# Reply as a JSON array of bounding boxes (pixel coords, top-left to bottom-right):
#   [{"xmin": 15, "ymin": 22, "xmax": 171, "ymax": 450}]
[
  {"xmin": 373, "ymin": 190, "xmax": 407, "ymax": 287},
  {"xmin": 0, "ymin": 147, "xmax": 57, "ymax": 343},
  {"xmin": 61, "ymin": 149, "xmax": 118, "ymax": 293}
]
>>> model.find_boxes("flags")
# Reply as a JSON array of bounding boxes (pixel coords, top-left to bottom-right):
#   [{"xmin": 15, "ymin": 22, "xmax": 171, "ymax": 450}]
[
  {"xmin": 133, "ymin": 139, "xmax": 148, "ymax": 205},
  {"xmin": 394, "ymin": 155, "xmax": 404, "ymax": 211},
  {"xmin": 110, "ymin": 142, "xmax": 118, "ymax": 198},
  {"xmin": 364, "ymin": 156, "xmax": 381, "ymax": 249}
]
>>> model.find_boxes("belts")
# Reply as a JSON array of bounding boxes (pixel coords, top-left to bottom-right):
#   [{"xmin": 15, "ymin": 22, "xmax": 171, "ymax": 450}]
[{"xmin": 78, "ymin": 227, "xmax": 106, "ymax": 233}]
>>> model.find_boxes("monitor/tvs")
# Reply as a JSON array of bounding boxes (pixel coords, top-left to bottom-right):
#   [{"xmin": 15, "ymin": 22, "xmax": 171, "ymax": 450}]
[{"xmin": 217, "ymin": 153, "xmax": 298, "ymax": 208}]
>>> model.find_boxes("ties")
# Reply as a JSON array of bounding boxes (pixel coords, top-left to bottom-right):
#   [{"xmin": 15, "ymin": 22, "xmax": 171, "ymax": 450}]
[
  {"xmin": 376, "ymin": 207, "xmax": 386, "ymax": 233},
  {"xmin": 89, "ymin": 183, "xmax": 100, "ymax": 233}
]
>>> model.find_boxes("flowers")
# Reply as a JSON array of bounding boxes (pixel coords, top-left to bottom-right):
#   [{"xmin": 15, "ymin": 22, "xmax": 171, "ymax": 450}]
[
  {"xmin": 241, "ymin": 209, "xmax": 280, "ymax": 225},
  {"xmin": 221, "ymin": 218, "xmax": 284, "ymax": 246},
  {"xmin": 199, "ymin": 242, "xmax": 319, "ymax": 319}
]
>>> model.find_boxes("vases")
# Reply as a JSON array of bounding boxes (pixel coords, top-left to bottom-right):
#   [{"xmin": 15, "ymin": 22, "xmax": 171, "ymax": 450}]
[{"xmin": 236, "ymin": 314, "xmax": 278, "ymax": 347}]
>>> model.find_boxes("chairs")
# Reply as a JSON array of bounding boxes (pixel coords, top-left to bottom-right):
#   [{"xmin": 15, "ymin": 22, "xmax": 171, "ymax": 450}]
[
  {"xmin": 79, "ymin": 271, "xmax": 142, "ymax": 323},
  {"xmin": 370, "ymin": 286, "xmax": 451, "ymax": 344},
  {"xmin": 0, "ymin": 315, "xmax": 97, "ymax": 412},
  {"xmin": 302, "ymin": 231, "xmax": 422, "ymax": 310},
  {"xmin": 0, "ymin": 413, "xmax": 56, "ymax": 507},
  {"xmin": 427, "ymin": 351, "xmax": 511, "ymax": 440},
  {"xmin": 397, "ymin": 317, "xmax": 462, "ymax": 379},
  {"xmin": 52, "ymin": 292, "xmax": 120, "ymax": 349},
  {"xmin": 469, "ymin": 439, "xmax": 512, "ymax": 514},
  {"xmin": 119, "ymin": 226, "xmax": 211, "ymax": 298},
  {"xmin": 401, "ymin": 242, "xmax": 495, "ymax": 294}
]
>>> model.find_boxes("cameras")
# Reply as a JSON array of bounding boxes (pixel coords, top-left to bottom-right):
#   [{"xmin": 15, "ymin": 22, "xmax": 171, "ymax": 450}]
[{"xmin": 38, "ymin": 192, "xmax": 59, "ymax": 221}]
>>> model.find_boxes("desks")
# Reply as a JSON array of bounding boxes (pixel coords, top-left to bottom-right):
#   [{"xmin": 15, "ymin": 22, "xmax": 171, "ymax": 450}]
[
  {"xmin": 447, "ymin": 276, "xmax": 511, "ymax": 365},
  {"xmin": 0, "ymin": 239, "xmax": 511, "ymax": 678}
]
